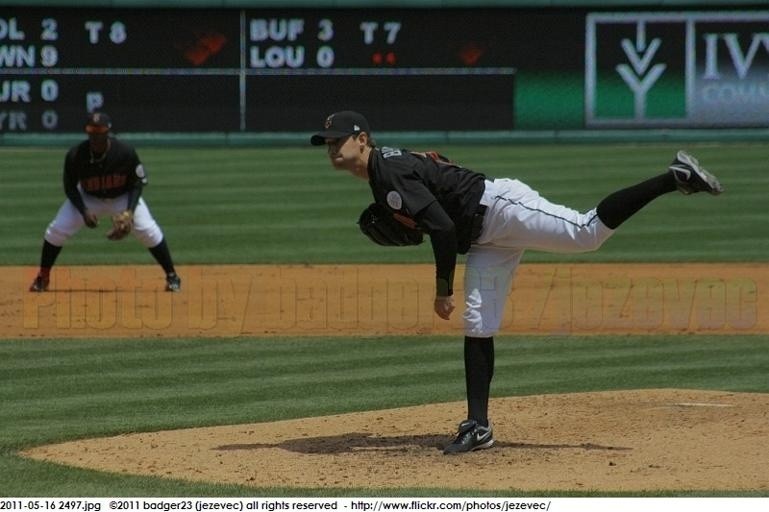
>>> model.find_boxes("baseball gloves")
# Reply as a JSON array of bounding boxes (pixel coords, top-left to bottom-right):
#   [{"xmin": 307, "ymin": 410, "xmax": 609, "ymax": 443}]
[
  {"xmin": 358, "ymin": 203, "xmax": 423, "ymax": 246},
  {"xmin": 107, "ymin": 211, "xmax": 135, "ymax": 238}
]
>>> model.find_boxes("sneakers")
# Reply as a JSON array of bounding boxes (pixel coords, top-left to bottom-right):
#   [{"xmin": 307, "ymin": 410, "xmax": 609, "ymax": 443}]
[
  {"xmin": 443, "ymin": 420, "xmax": 495, "ymax": 456},
  {"xmin": 668, "ymin": 150, "xmax": 725, "ymax": 196},
  {"xmin": 165, "ymin": 274, "xmax": 181, "ymax": 292},
  {"xmin": 29, "ymin": 271, "xmax": 50, "ymax": 292}
]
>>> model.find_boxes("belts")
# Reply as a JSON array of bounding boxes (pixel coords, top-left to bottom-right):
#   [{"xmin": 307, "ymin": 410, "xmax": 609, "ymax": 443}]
[{"xmin": 468, "ymin": 176, "xmax": 497, "ymax": 243}]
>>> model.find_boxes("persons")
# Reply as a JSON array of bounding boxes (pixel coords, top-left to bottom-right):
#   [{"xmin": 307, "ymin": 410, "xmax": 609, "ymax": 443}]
[
  {"xmin": 311, "ymin": 111, "xmax": 723, "ymax": 456},
  {"xmin": 29, "ymin": 112, "xmax": 181, "ymax": 292}
]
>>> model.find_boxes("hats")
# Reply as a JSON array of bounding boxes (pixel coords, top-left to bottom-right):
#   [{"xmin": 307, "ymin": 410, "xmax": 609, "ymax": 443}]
[
  {"xmin": 310, "ymin": 110, "xmax": 369, "ymax": 146},
  {"xmin": 83, "ymin": 111, "xmax": 112, "ymax": 129}
]
